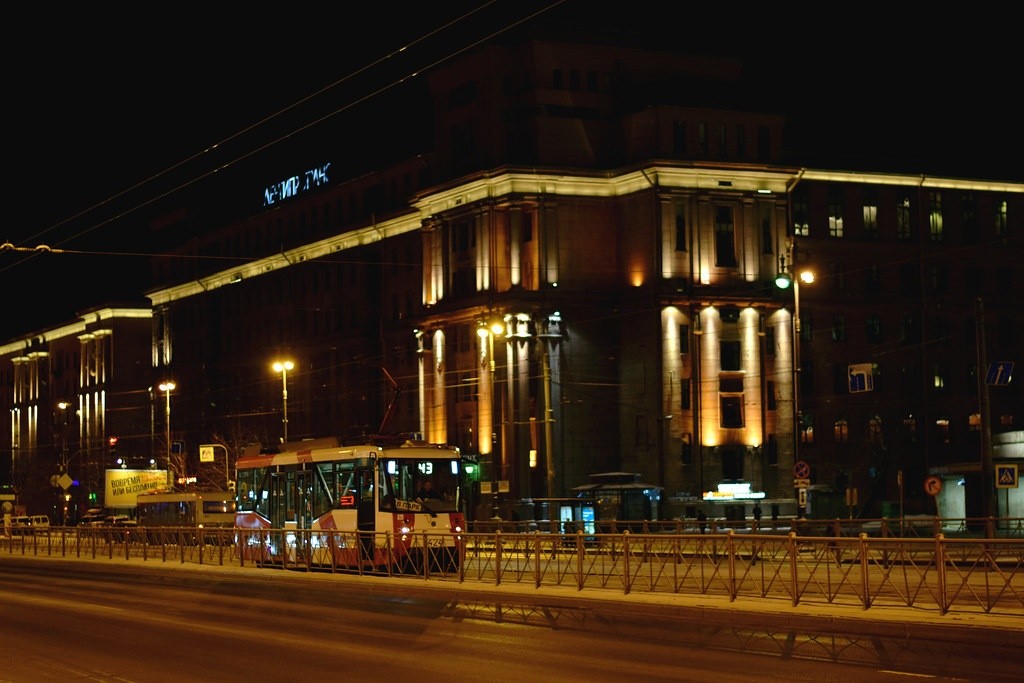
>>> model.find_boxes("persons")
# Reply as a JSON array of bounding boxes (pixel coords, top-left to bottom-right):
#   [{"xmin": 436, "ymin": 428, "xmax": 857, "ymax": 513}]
[
  {"xmin": 696, "ymin": 509, "xmax": 707, "ymax": 534},
  {"xmin": 752, "ymin": 503, "xmax": 762, "ymax": 530},
  {"xmin": 416, "ymin": 480, "xmax": 442, "ymax": 500}
]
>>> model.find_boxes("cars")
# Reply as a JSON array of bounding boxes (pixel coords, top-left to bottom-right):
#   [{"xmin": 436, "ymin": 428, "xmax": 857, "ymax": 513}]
[{"xmin": 77, "ymin": 508, "xmax": 136, "ymax": 543}]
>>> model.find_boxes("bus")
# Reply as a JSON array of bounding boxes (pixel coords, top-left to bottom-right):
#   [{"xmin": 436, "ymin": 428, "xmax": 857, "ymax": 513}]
[
  {"xmin": 137, "ymin": 491, "xmax": 235, "ymax": 545},
  {"xmin": 233, "ymin": 440, "xmax": 472, "ymax": 576}
]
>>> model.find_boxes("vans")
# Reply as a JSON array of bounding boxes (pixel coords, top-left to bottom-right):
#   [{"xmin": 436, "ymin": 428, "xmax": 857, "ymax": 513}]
[{"xmin": 0, "ymin": 515, "xmax": 51, "ymax": 535}]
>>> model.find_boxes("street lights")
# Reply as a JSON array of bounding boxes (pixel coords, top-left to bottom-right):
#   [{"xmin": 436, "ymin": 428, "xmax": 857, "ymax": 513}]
[
  {"xmin": 58, "ymin": 402, "xmax": 68, "ymax": 529},
  {"xmin": 272, "ymin": 360, "xmax": 296, "ymax": 443},
  {"xmin": 477, "ymin": 323, "xmax": 504, "ymax": 523},
  {"xmin": 159, "ymin": 382, "xmax": 174, "ymax": 486},
  {"xmin": 792, "ymin": 271, "xmax": 815, "ymax": 518}
]
